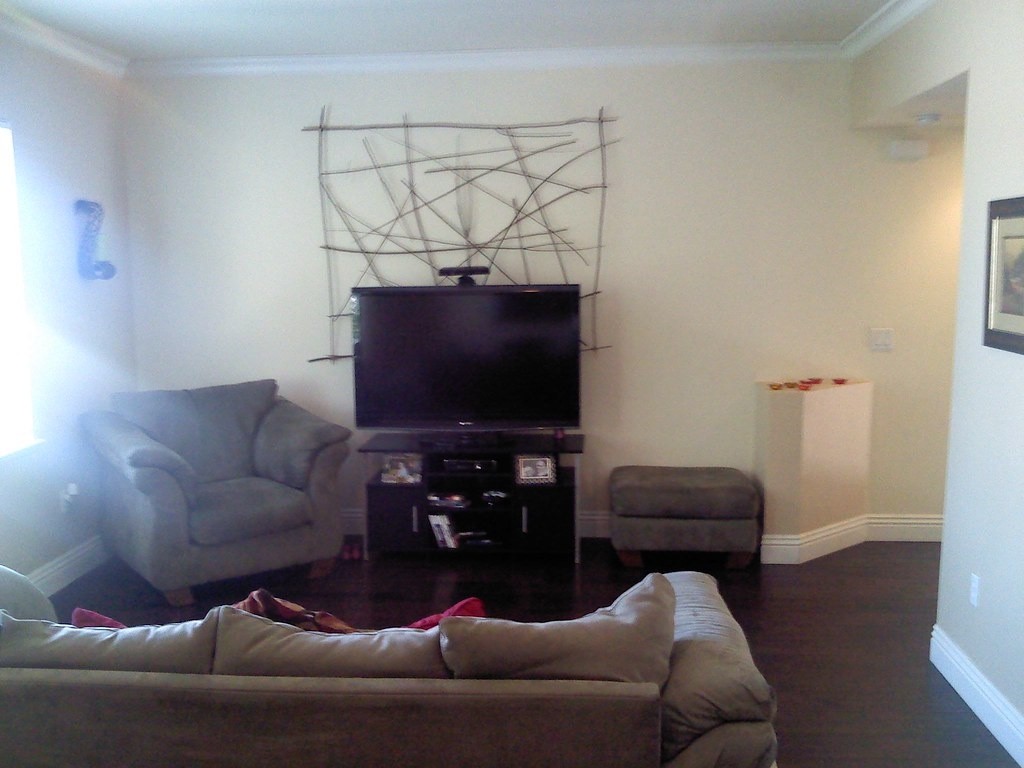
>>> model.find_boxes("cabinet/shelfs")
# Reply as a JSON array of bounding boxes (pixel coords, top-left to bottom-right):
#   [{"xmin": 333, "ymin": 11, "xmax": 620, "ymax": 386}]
[{"xmin": 357, "ymin": 433, "xmax": 586, "ymax": 561}]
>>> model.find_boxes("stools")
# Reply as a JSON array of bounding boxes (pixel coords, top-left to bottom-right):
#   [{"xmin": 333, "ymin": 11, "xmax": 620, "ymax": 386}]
[{"xmin": 608, "ymin": 465, "xmax": 760, "ymax": 571}]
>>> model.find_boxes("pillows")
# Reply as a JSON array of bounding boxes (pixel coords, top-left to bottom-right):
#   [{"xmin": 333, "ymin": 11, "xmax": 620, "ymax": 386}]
[
  {"xmin": 214, "ymin": 604, "xmax": 452, "ymax": 680},
  {"xmin": 278, "ymin": 597, "xmax": 485, "ymax": 633},
  {"xmin": 71, "ymin": 589, "xmax": 274, "ymax": 629},
  {"xmin": 440, "ymin": 572, "xmax": 677, "ymax": 692},
  {"xmin": 0, "ymin": 606, "xmax": 222, "ymax": 673}
]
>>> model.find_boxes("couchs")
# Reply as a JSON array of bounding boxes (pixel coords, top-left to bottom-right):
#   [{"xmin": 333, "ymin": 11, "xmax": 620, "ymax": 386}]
[
  {"xmin": 0, "ymin": 572, "xmax": 780, "ymax": 768},
  {"xmin": 83, "ymin": 378, "xmax": 353, "ymax": 609}
]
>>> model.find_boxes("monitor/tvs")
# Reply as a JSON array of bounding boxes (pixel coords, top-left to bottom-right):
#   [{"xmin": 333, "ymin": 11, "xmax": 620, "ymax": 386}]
[{"xmin": 353, "ymin": 284, "xmax": 581, "ymax": 448}]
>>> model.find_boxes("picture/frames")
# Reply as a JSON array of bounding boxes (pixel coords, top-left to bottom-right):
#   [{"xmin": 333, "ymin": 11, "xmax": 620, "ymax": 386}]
[{"xmin": 981, "ymin": 197, "xmax": 1024, "ymax": 355}]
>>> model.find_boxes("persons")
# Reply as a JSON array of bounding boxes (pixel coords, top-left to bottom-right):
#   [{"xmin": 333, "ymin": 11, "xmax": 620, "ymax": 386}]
[
  {"xmin": 398, "ymin": 462, "xmax": 407, "ymax": 477},
  {"xmin": 536, "ymin": 462, "xmax": 548, "ymax": 476}
]
